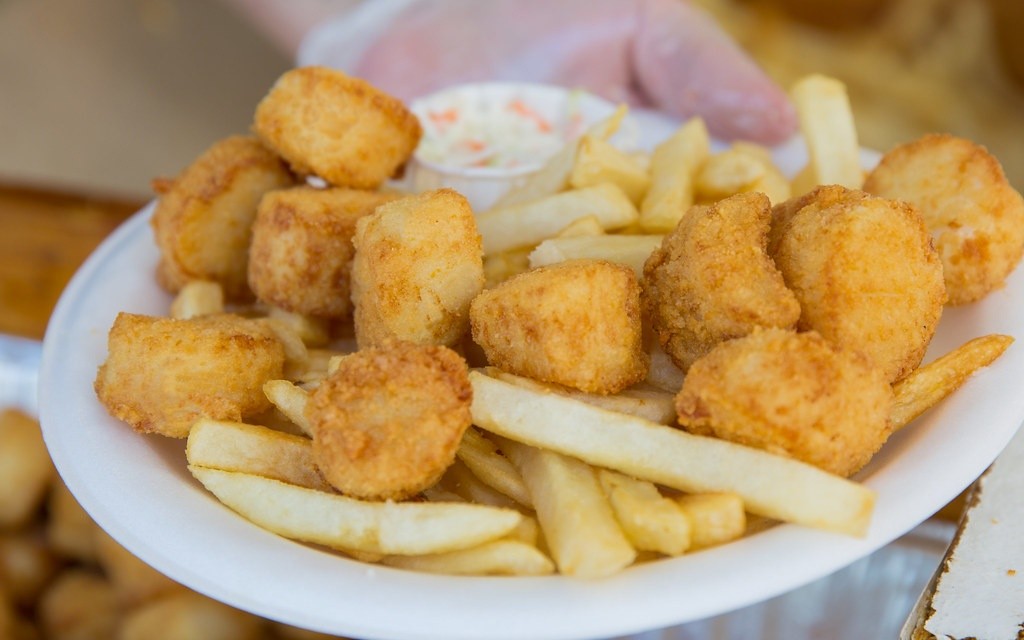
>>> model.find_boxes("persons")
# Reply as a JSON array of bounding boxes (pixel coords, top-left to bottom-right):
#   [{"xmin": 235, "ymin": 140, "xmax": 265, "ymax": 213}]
[{"xmin": 245, "ymin": 0, "xmax": 798, "ymax": 149}]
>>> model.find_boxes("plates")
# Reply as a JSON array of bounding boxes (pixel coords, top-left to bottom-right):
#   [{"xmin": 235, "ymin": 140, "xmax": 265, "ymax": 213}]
[{"xmin": 38, "ymin": 100, "xmax": 1024, "ymax": 640}]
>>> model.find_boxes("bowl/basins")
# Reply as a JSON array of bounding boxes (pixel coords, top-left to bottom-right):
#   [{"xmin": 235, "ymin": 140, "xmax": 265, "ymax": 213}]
[{"xmin": 406, "ymin": 80, "xmax": 634, "ymax": 210}]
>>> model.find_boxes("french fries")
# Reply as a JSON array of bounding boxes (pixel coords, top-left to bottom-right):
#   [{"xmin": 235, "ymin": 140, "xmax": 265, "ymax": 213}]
[{"xmin": 175, "ymin": 74, "xmax": 1015, "ymax": 575}]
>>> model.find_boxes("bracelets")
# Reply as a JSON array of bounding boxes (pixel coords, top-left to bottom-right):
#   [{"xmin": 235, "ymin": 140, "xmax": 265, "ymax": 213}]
[{"xmin": 294, "ymin": 0, "xmax": 409, "ymax": 79}]
